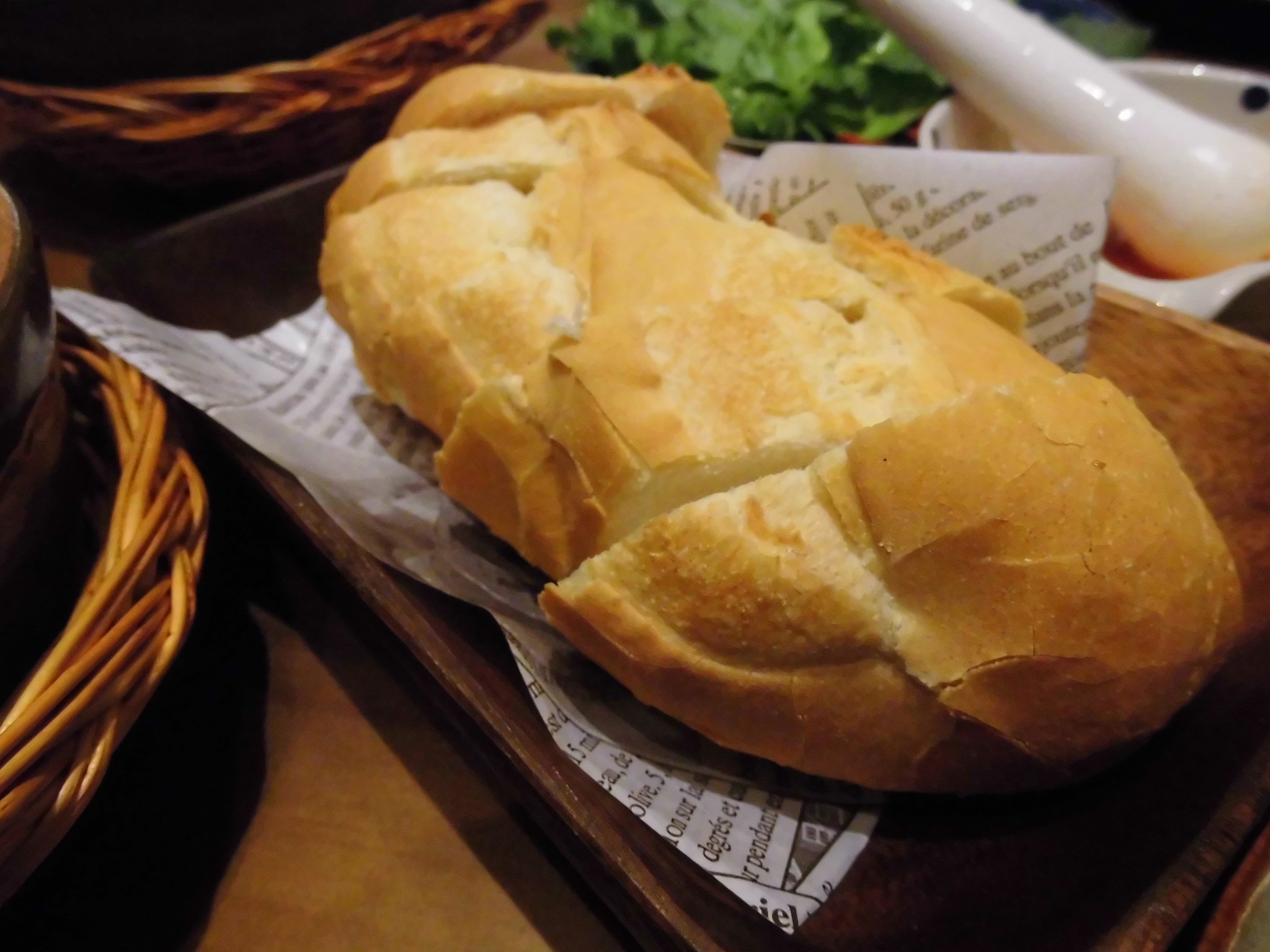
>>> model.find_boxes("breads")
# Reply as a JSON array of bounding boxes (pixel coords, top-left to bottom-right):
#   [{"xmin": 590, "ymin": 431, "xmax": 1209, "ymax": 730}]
[{"xmin": 320, "ymin": 63, "xmax": 1246, "ymax": 795}]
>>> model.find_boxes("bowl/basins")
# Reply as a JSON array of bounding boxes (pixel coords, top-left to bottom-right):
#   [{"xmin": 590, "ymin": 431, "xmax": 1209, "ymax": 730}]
[{"xmin": 924, "ymin": 62, "xmax": 1269, "ymax": 330}]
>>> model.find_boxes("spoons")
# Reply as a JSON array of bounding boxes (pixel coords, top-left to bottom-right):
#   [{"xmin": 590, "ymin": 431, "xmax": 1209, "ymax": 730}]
[{"xmin": 854, "ymin": 0, "xmax": 1270, "ymax": 279}]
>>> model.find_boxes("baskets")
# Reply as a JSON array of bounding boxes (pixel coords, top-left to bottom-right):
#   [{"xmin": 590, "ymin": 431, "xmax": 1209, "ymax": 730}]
[
  {"xmin": 0, "ymin": 0, "xmax": 547, "ymax": 215},
  {"xmin": 1, "ymin": 316, "xmax": 211, "ymax": 901}
]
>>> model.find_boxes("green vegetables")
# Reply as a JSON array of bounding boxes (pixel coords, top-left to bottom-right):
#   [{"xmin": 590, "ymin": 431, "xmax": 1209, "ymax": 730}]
[{"xmin": 545, "ymin": 0, "xmax": 950, "ymax": 144}]
